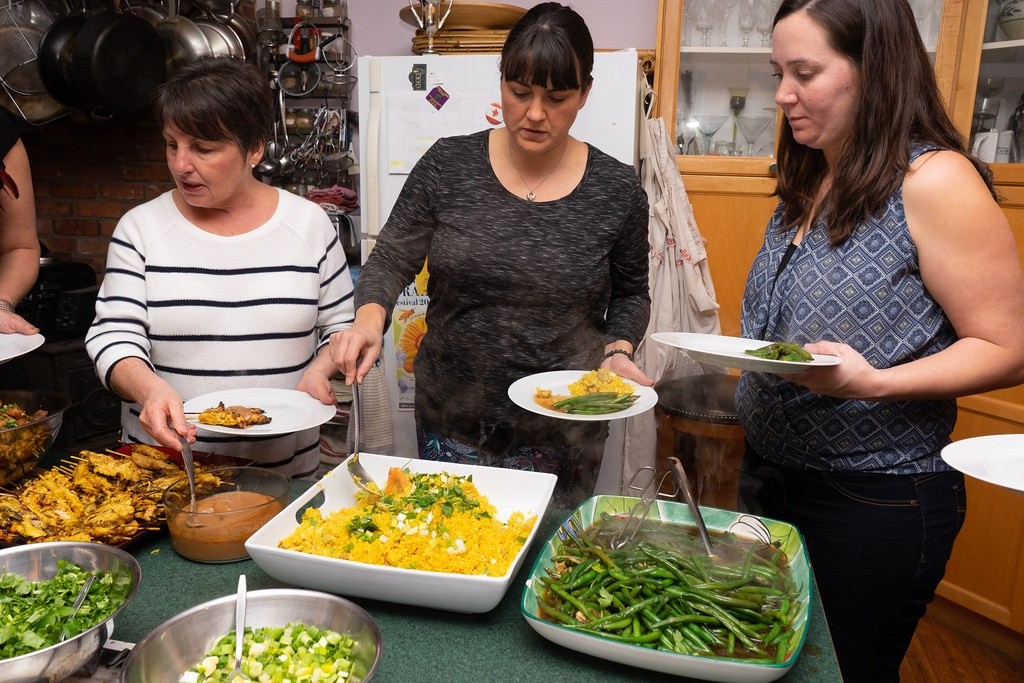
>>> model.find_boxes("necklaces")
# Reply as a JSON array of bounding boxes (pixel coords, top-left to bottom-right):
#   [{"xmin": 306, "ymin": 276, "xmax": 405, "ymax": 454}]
[{"xmin": 505, "ymin": 134, "xmax": 565, "ymax": 202}]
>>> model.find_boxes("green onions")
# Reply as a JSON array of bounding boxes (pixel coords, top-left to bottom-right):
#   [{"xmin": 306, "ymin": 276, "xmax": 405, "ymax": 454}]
[
  {"xmin": 181, "ymin": 623, "xmax": 353, "ymax": 683},
  {"xmin": 0, "ymin": 402, "xmax": 21, "ymax": 429},
  {"xmin": 344, "ymin": 474, "xmax": 528, "ymax": 553}
]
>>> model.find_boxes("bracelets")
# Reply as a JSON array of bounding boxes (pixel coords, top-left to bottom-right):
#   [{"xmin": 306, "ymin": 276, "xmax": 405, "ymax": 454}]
[
  {"xmin": 599, "ymin": 349, "xmax": 634, "ymax": 361},
  {"xmin": 0, "ymin": 299, "xmax": 16, "ymax": 313}
]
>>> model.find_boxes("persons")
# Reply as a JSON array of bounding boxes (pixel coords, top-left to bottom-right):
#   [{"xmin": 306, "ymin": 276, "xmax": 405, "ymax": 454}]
[
  {"xmin": 731, "ymin": 0, "xmax": 1024, "ymax": 683},
  {"xmin": 0, "ymin": 103, "xmax": 44, "ymax": 339},
  {"xmin": 80, "ymin": 56, "xmax": 357, "ymax": 482},
  {"xmin": 329, "ymin": 0, "xmax": 655, "ymax": 510}
]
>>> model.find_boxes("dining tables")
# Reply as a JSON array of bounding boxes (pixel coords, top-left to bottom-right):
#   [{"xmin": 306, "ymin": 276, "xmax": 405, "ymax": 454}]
[{"xmin": 105, "ymin": 476, "xmax": 843, "ymax": 683}]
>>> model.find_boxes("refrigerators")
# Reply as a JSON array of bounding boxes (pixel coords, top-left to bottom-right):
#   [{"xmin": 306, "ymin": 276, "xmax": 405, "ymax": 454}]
[{"xmin": 357, "ymin": 46, "xmax": 643, "ymax": 493}]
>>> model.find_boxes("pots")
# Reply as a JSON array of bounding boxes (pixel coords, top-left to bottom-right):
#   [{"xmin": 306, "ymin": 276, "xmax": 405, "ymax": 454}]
[{"xmin": 0, "ymin": 0, "xmax": 258, "ymax": 126}]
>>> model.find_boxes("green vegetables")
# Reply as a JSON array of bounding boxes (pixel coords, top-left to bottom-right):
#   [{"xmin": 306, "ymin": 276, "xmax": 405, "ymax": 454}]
[{"xmin": 0, "ymin": 559, "xmax": 126, "ymax": 660}]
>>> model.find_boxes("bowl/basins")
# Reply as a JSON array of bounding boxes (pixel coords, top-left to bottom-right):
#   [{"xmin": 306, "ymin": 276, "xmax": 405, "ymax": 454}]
[
  {"xmin": 244, "ymin": 451, "xmax": 558, "ymax": 614},
  {"xmin": 0, "ymin": 541, "xmax": 142, "ymax": 683},
  {"xmin": 162, "ymin": 466, "xmax": 291, "ymax": 563},
  {"xmin": 346, "ymin": 357, "xmax": 395, "ymax": 458},
  {"xmin": 118, "ymin": 588, "xmax": 382, "ymax": 683},
  {"xmin": 0, "ymin": 387, "xmax": 72, "ymax": 489}
]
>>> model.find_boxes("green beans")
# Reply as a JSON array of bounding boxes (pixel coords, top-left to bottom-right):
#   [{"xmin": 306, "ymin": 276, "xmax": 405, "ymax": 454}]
[
  {"xmin": 744, "ymin": 342, "xmax": 814, "ymax": 362},
  {"xmin": 536, "ymin": 511, "xmax": 802, "ymax": 664},
  {"xmin": 552, "ymin": 392, "xmax": 640, "ymax": 414}
]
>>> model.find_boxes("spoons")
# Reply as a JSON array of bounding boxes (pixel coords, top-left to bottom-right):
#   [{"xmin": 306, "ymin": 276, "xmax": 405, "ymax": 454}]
[
  {"xmin": 170, "ymin": 426, "xmax": 206, "ymax": 527},
  {"xmin": 222, "ymin": 575, "xmax": 253, "ymax": 683},
  {"xmin": 677, "ymin": 135, "xmax": 695, "ymax": 155}
]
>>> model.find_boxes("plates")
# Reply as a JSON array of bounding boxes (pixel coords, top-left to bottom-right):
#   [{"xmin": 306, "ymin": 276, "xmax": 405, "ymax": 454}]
[
  {"xmin": 941, "ymin": 433, "xmax": 1024, "ymax": 496},
  {"xmin": 520, "ymin": 493, "xmax": 814, "ymax": 683},
  {"xmin": 507, "ymin": 370, "xmax": 659, "ymax": 421},
  {"xmin": 0, "ymin": 331, "xmax": 45, "ymax": 365},
  {"xmin": 651, "ymin": 331, "xmax": 842, "ymax": 375},
  {"xmin": 182, "ymin": 387, "xmax": 336, "ymax": 437}
]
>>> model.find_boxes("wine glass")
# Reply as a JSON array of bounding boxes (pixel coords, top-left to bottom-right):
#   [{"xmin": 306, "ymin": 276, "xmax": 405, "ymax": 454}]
[
  {"xmin": 970, "ymin": 72, "xmax": 1005, "ymax": 137},
  {"xmin": 909, "ymin": 0, "xmax": 946, "ymax": 53},
  {"xmin": 693, "ymin": 86, "xmax": 778, "ymax": 158},
  {"xmin": 681, "ymin": 0, "xmax": 780, "ymax": 48}
]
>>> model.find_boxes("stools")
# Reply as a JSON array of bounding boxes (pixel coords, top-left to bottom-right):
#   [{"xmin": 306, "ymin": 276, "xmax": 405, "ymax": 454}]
[{"xmin": 660, "ymin": 413, "xmax": 744, "ymax": 512}]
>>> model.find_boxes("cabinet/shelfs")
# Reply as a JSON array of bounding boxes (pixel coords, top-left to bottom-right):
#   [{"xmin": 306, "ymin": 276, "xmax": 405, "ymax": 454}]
[{"xmin": 655, "ymin": 0, "xmax": 1024, "ymax": 634}]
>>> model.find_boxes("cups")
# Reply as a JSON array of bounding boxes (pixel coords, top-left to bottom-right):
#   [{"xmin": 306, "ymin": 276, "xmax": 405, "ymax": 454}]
[{"xmin": 971, "ymin": 132, "xmax": 999, "ymax": 163}]
